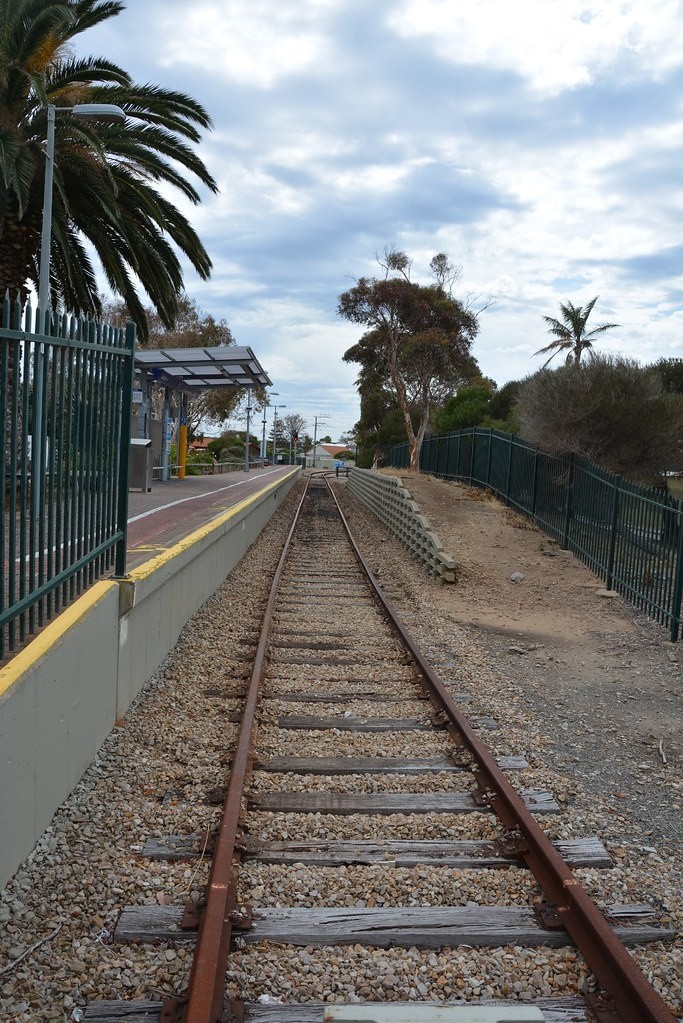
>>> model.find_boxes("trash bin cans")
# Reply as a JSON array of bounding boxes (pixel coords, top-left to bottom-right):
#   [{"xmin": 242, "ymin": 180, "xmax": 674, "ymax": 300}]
[{"xmin": 129, "ymin": 438, "xmax": 154, "ymax": 495}]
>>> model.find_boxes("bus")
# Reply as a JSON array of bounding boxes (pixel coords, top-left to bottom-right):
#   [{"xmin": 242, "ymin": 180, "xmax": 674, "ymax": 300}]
[{"xmin": 254, "ymin": 459, "xmax": 269, "ymax": 466}]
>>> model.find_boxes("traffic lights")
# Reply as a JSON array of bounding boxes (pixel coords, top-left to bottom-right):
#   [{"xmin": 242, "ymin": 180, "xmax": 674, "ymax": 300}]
[{"xmin": 294, "ymin": 432, "xmax": 298, "ymax": 441}]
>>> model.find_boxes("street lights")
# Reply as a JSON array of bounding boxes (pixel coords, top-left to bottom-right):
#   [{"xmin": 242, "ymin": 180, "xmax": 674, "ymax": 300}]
[
  {"xmin": 272, "ymin": 405, "xmax": 287, "ymax": 465},
  {"xmin": 261, "ymin": 391, "xmax": 280, "ymax": 467},
  {"xmin": 27, "ymin": 99, "xmax": 131, "ymax": 523}
]
